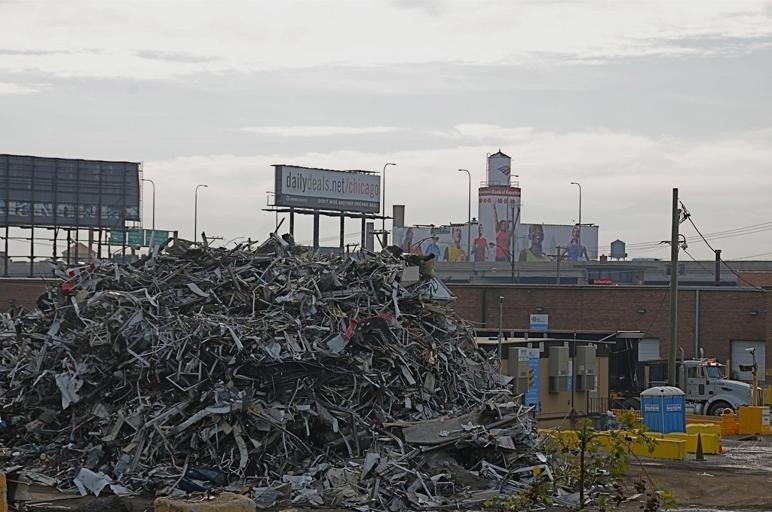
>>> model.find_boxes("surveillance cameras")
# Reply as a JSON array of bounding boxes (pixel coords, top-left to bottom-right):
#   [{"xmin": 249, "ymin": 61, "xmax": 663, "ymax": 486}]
[
  {"xmin": 682, "ymin": 244, "xmax": 688, "ymax": 250},
  {"xmin": 744, "ymin": 347, "xmax": 755, "ymax": 352}
]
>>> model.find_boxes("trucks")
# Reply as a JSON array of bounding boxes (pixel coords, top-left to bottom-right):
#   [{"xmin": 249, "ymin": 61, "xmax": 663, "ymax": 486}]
[{"xmin": 472, "ymin": 328, "xmax": 763, "ymax": 416}]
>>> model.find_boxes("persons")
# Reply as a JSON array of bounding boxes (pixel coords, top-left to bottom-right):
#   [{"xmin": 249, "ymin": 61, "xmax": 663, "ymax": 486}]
[
  {"xmin": 401, "ymin": 221, "xmax": 514, "ymax": 263},
  {"xmin": 519, "ymin": 225, "xmax": 551, "ymax": 261},
  {"xmin": 558, "ymin": 226, "xmax": 590, "ymax": 262}
]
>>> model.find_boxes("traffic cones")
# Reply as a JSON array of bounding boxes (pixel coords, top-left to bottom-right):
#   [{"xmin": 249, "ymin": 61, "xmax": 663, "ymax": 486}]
[{"xmin": 693, "ymin": 432, "xmax": 707, "ymax": 461}]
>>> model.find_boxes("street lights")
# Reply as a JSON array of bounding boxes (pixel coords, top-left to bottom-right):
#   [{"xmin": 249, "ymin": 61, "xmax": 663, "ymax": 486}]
[
  {"xmin": 383, "ymin": 162, "xmax": 396, "ymax": 231},
  {"xmin": 570, "ymin": 182, "xmax": 581, "ymax": 246},
  {"xmin": 407, "ymin": 236, "xmax": 440, "ymax": 253},
  {"xmin": 489, "ymin": 175, "xmax": 520, "ymax": 283},
  {"xmin": 458, "ymin": 169, "xmax": 470, "ymax": 261},
  {"xmin": 195, "ymin": 184, "xmax": 208, "ymax": 248},
  {"xmin": 547, "ymin": 246, "xmax": 569, "ymax": 284},
  {"xmin": 266, "ymin": 191, "xmax": 278, "ymax": 235},
  {"xmin": 140, "ymin": 178, "xmax": 155, "ymax": 230}
]
